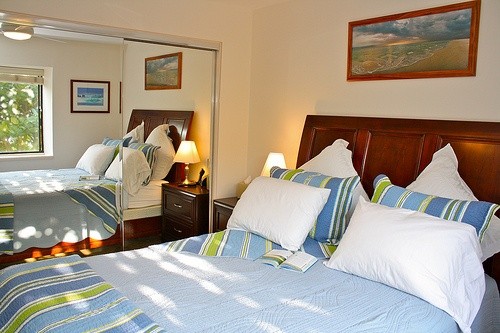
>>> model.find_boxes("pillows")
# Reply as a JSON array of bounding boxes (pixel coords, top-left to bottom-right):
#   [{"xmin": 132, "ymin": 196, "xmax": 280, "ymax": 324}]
[
  {"xmin": 74, "ymin": 120, "xmax": 175, "ymax": 196},
  {"xmin": 227, "ymin": 137, "xmax": 500, "ymax": 333}
]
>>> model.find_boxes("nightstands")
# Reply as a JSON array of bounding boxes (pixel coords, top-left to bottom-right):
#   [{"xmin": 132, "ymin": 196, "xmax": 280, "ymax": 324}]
[
  {"xmin": 212, "ymin": 197, "xmax": 240, "ymax": 232},
  {"xmin": 160, "ymin": 182, "xmax": 209, "ymax": 242}
]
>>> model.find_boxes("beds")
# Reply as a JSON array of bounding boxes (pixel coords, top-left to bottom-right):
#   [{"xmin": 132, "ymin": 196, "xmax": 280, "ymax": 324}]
[
  {"xmin": 0, "ymin": 114, "xmax": 500, "ymax": 333},
  {"xmin": 0, "ymin": 109, "xmax": 194, "ymax": 266}
]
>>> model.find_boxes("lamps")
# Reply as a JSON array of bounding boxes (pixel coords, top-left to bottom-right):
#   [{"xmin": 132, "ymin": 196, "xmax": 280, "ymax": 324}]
[
  {"xmin": 1, "ymin": 24, "xmax": 35, "ymax": 41},
  {"xmin": 261, "ymin": 152, "xmax": 286, "ymax": 178},
  {"xmin": 173, "ymin": 140, "xmax": 201, "ymax": 188}
]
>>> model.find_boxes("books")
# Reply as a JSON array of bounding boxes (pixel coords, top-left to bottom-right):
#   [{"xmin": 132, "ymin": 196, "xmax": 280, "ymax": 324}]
[
  {"xmin": 79, "ymin": 175, "xmax": 100, "ymax": 181},
  {"xmin": 253, "ymin": 249, "xmax": 319, "ymax": 274}
]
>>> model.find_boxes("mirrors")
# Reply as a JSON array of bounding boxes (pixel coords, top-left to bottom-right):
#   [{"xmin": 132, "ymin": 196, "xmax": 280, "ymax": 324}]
[{"xmin": 0, "ymin": 10, "xmax": 223, "ymax": 269}]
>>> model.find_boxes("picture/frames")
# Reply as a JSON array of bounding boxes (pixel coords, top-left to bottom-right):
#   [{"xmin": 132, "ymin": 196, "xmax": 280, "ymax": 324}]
[
  {"xmin": 346, "ymin": 0, "xmax": 481, "ymax": 82},
  {"xmin": 144, "ymin": 52, "xmax": 182, "ymax": 90},
  {"xmin": 70, "ymin": 79, "xmax": 110, "ymax": 113}
]
